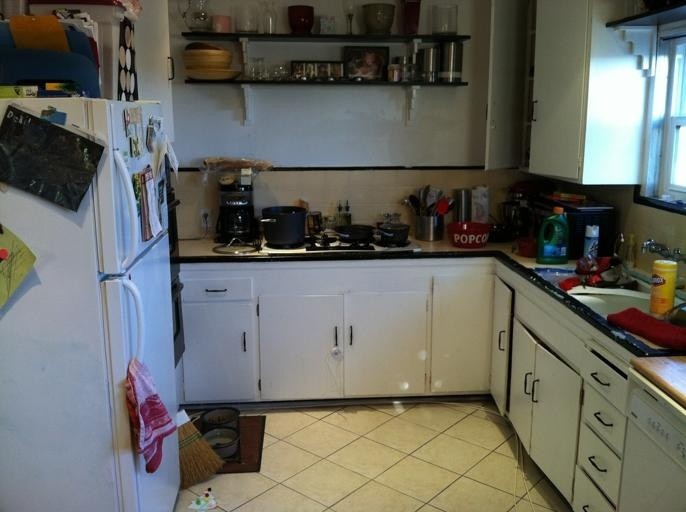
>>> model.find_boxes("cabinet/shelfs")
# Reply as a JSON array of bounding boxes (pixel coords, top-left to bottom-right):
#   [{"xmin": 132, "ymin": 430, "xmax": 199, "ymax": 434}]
[
  {"xmin": 260, "ymin": 292, "xmax": 430, "ymax": 410},
  {"xmin": 571, "ymin": 338, "xmax": 630, "ymax": 512},
  {"xmin": 508, "ymin": 317, "xmax": 584, "ymax": 507},
  {"xmin": 181, "ymin": 264, "xmax": 258, "ymax": 411},
  {"xmin": 491, "ymin": 273, "xmax": 517, "ymax": 418},
  {"xmin": 180, "ymin": 31, "xmax": 471, "ymax": 127},
  {"xmin": 429, "ymin": 273, "xmax": 495, "ymax": 404},
  {"xmin": 484, "ymin": 1, "xmax": 654, "ymax": 187}
]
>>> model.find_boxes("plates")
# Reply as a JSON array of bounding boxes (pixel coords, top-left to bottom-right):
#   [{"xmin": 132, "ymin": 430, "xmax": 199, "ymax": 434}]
[{"xmin": 181, "ymin": 68, "xmax": 242, "ymax": 80}]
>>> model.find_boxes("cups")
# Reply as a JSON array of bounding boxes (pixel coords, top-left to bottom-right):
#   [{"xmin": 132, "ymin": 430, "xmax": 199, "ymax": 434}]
[
  {"xmin": 431, "ymin": 4, "xmax": 459, "ymax": 36},
  {"xmin": 235, "ymin": 5, "xmax": 259, "ymax": 33}
]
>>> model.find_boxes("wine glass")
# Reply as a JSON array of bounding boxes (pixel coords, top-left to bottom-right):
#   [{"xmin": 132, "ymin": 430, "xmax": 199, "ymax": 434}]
[{"xmin": 343, "ymin": 0, "xmax": 356, "ymax": 35}]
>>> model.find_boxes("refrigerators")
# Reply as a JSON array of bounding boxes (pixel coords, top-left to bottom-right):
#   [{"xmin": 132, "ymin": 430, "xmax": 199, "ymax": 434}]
[{"xmin": 1, "ymin": 96, "xmax": 181, "ymax": 511}]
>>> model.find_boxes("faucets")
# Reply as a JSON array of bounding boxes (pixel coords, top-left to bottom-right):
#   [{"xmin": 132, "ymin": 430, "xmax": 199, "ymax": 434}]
[
  {"xmin": 613, "ymin": 231, "xmax": 627, "ymax": 263},
  {"xmin": 642, "ymin": 237, "xmax": 684, "ymax": 261}
]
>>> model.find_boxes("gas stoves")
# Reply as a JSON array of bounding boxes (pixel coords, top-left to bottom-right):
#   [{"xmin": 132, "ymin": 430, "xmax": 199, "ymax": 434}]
[{"xmin": 262, "ymin": 223, "xmax": 412, "ymax": 254}]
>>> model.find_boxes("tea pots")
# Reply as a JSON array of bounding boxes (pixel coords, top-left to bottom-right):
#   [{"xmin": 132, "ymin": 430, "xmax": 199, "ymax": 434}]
[{"xmin": 176, "ymin": 0, "xmax": 214, "ymax": 33}]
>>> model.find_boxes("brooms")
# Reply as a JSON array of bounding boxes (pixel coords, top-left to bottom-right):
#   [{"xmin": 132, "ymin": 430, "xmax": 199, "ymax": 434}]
[{"xmin": 176, "ymin": 407, "xmax": 226, "ymax": 490}]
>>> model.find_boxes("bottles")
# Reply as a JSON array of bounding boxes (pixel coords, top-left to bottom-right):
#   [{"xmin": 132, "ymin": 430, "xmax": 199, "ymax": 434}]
[
  {"xmin": 649, "ymin": 259, "xmax": 677, "ymax": 319},
  {"xmin": 263, "ymin": 1, "xmax": 278, "ymax": 36},
  {"xmin": 389, "ymin": 37, "xmax": 424, "ymax": 83},
  {"xmin": 582, "ymin": 224, "xmax": 600, "ymax": 261},
  {"xmin": 336, "ymin": 200, "xmax": 352, "ymax": 227},
  {"xmin": 250, "ymin": 57, "xmax": 289, "ymax": 82}
]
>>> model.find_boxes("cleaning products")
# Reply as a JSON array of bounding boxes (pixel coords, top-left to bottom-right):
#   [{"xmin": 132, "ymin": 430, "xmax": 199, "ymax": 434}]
[{"xmin": 625, "ymin": 233, "xmax": 637, "ymax": 267}]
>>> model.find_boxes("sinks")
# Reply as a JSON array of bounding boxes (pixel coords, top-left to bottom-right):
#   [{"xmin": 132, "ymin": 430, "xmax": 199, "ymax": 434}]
[
  {"xmin": 534, "ymin": 266, "xmax": 657, "ymax": 294},
  {"xmin": 570, "ymin": 292, "xmax": 686, "ymax": 350}
]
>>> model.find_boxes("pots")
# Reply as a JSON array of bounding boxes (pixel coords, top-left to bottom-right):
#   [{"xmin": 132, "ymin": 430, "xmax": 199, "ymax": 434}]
[
  {"xmin": 261, "ymin": 206, "xmax": 307, "ymax": 246},
  {"xmin": 349, "ymin": 225, "xmax": 375, "ymax": 243},
  {"xmin": 377, "ymin": 224, "xmax": 411, "ymax": 246}
]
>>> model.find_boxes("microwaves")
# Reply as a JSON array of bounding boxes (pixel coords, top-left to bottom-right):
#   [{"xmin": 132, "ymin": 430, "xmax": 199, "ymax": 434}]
[{"xmin": 528, "ymin": 195, "xmax": 618, "ymax": 260}]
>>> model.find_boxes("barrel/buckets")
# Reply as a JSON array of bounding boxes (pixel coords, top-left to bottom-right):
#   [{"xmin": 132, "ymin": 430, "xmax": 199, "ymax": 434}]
[{"xmin": 537, "ymin": 206, "xmax": 569, "ymax": 264}]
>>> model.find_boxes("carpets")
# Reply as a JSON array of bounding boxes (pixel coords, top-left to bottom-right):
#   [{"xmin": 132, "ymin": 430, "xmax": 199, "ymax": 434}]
[{"xmin": 194, "ymin": 414, "xmax": 267, "ymax": 473}]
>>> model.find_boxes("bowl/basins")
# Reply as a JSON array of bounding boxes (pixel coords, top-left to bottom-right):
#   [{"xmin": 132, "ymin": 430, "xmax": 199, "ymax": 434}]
[
  {"xmin": 199, "ymin": 407, "xmax": 240, "ymax": 428},
  {"xmin": 182, "ymin": 49, "xmax": 235, "ymax": 69},
  {"xmin": 200, "ymin": 426, "xmax": 240, "ymax": 459},
  {"xmin": 447, "ymin": 221, "xmax": 490, "ymax": 249}
]
